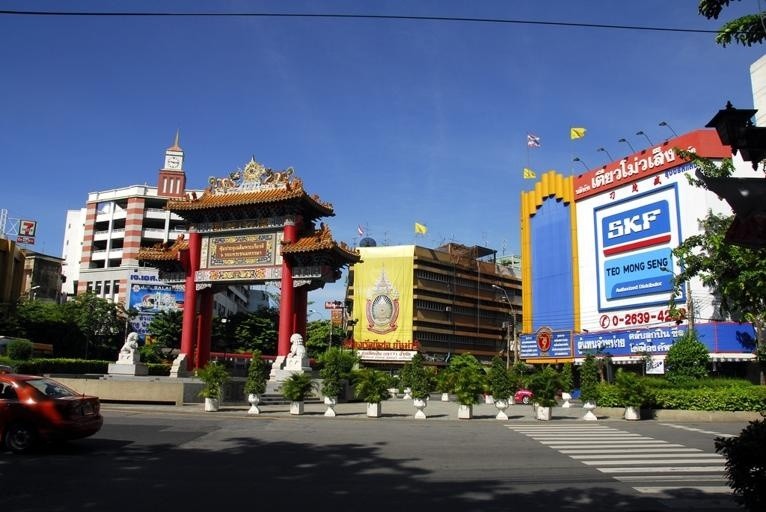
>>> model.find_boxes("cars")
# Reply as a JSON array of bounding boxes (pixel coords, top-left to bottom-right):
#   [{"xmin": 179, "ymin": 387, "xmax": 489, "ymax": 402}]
[
  {"xmin": 0, "ymin": 373, "xmax": 104, "ymax": 455},
  {"xmin": 483, "ymin": 383, "xmax": 534, "ymax": 405}
]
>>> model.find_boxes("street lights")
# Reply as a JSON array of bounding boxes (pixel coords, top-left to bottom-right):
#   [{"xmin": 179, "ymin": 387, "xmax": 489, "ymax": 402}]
[
  {"xmin": 604, "ymin": 352, "xmax": 614, "ymax": 381},
  {"xmin": 15, "ymin": 286, "xmax": 41, "ymax": 304},
  {"xmin": 219, "ymin": 316, "xmax": 227, "ymax": 352},
  {"xmin": 490, "ymin": 283, "xmax": 517, "ymax": 368},
  {"xmin": 660, "ymin": 265, "xmax": 690, "ymax": 334},
  {"xmin": 307, "ymin": 308, "xmax": 332, "ymax": 349}
]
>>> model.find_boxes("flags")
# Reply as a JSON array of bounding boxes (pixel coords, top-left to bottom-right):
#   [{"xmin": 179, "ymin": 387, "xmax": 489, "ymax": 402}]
[
  {"xmin": 358, "ymin": 224, "xmax": 364, "ymax": 240},
  {"xmin": 527, "ymin": 133, "xmax": 543, "ymax": 150},
  {"xmin": 522, "ymin": 168, "xmax": 537, "ymax": 179},
  {"xmin": 570, "ymin": 127, "xmax": 587, "ymax": 141},
  {"xmin": 415, "ymin": 222, "xmax": 428, "ymax": 235}
]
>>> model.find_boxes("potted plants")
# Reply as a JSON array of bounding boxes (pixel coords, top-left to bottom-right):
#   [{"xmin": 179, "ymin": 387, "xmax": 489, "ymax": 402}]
[
  {"xmin": 404, "ymin": 349, "xmax": 433, "ymax": 419},
  {"xmin": 190, "ymin": 353, "xmax": 233, "ymax": 416},
  {"xmin": 354, "ymin": 374, "xmax": 390, "ymax": 418},
  {"xmin": 524, "ymin": 367, "xmax": 559, "ymax": 422},
  {"xmin": 447, "ymin": 351, "xmax": 484, "ymax": 421},
  {"xmin": 315, "ymin": 349, "xmax": 348, "ymax": 418},
  {"xmin": 274, "ymin": 367, "xmax": 321, "ymax": 418},
  {"xmin": 484, "ymin": 353, "xmax": 519, "ymax": 421},
  {"xmin": 610, "ymin": 367, "xmax": 652, "ymax": 420},
  {"xmin": 556, "ymin": 359, "xmax": 577, "ymax": 408},
  {"xmin": 437, "ymin": 382, "xmax": 451, "ymax": 403},
  {"xmin": 574, "ymin": 351, "xmax": 601, "ymax": 423},
  {"xmin": 239, "ymin": 346, "xmax": 270, "ymax": 416}
]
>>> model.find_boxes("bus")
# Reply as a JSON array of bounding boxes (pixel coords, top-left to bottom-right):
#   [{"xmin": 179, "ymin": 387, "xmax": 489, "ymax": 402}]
[
  {"xmin": 194, "ymin": 350, "xmax": 317, "ymax": 370},
  {"xmin": 0, "ymin": 335, "xmax": 53, "ymax": 360}
]
